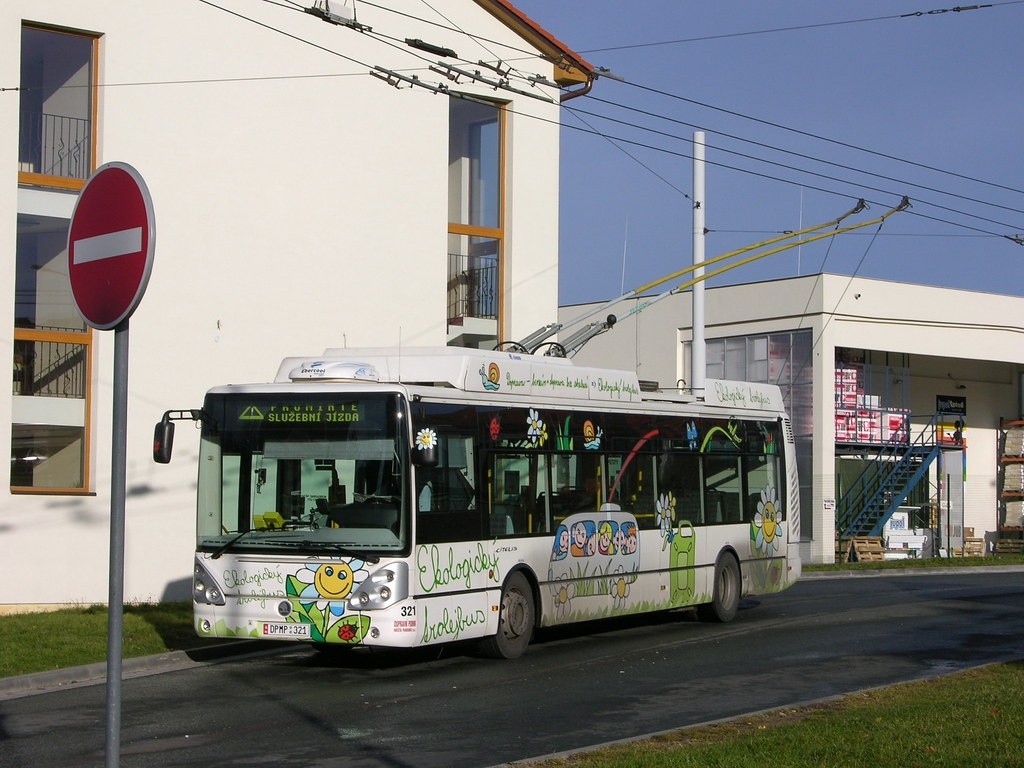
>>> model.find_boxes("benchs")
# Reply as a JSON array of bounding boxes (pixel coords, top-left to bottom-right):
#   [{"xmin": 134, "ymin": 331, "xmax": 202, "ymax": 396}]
[
  {"xmin": 991, "ymin": 539, "xmax": 1024, "ymax": 556},
  {"xmin": 950, "ymin": 538, "xmax": 984, "ymax": 557},
  {"xmin": 884, "ymin": 535, "xmax": 928, "ymax": 560}
]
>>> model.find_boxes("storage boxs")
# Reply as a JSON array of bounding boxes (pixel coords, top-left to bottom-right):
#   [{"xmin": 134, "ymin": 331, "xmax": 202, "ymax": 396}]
[{"xmin": 768, "ymin": 335, "xmax": 911, "ymax": 444}]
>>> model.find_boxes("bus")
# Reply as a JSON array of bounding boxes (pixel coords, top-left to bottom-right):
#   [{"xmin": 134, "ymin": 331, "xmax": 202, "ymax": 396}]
[{"xmin": 152, "ymin": 347, "xmax": 802, "ymax": 662}]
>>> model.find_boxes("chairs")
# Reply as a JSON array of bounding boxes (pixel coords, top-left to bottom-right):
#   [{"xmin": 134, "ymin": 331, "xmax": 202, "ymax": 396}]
[
  {"xmin": 538, "ymin": 486, "xmax": 584, "ymax": 502},
  {"xmin": 670, "ymin": 486, "xmax": 727, "ymax": 524}
]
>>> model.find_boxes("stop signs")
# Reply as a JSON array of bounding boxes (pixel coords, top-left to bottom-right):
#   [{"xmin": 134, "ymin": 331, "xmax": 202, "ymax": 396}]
[{"xmin": 66, "ymin": 161, "xmax": 157, "ymax": 332}]
